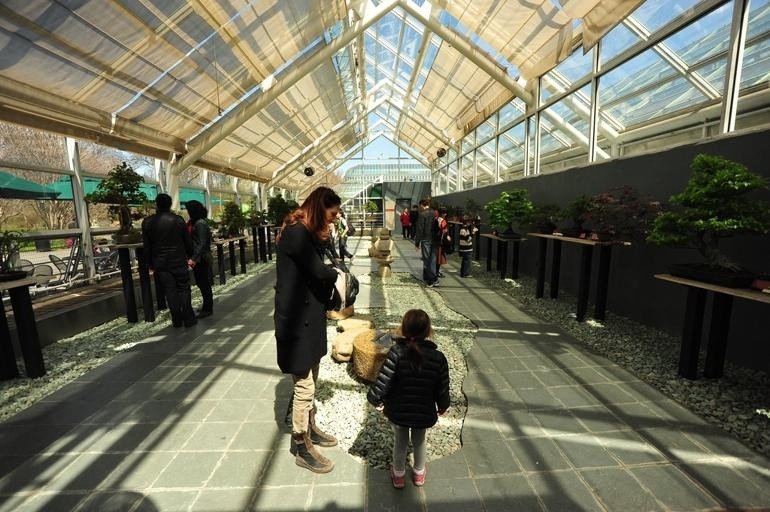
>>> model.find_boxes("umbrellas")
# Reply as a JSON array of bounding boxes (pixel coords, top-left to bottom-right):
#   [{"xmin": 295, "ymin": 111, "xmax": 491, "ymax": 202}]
[{"xmin": 0, "ymin": 171, "xmax": 227, "ymax": 210}]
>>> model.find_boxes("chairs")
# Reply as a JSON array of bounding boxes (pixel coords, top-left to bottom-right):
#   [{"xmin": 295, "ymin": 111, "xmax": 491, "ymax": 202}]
[{"xmin": 12, "ymin": 251, "xmax": 120, "ymax": 296}]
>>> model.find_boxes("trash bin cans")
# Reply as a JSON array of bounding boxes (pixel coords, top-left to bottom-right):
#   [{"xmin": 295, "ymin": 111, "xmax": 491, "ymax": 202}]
[{"xmin": 35, "ymin": 238, "xmax": 51, "ymax": 253}]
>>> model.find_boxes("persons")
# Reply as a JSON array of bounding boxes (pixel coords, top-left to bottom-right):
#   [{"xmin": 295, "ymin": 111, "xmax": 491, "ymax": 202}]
[
  {"xmin": 400, "ymin": 199, "xmax": 479, "ymax": 288},
  {"xmin": 273, "ymin": 187, "xmax": 359, "ymax": 474},
  {"xmin": 141, "ymin": 194, "xmax": 197, "ymax": 329},
  {"xmin": 67, "ymin": 216, "xmax": 78, "ymax": 248},
  {"xmin": 336, "ymin": 208, "xmax": 352, "ymax": 263},
  {"xmin": 319, "ymin": 223, "xmax": 339, "ymax": 262},
  {"xmin": 184, "ymin": 200, "xmax": 213, "ymax": 319},
  {"xmin": 366, "ymin": 310, "xmax": 451, "ymax": 489}
]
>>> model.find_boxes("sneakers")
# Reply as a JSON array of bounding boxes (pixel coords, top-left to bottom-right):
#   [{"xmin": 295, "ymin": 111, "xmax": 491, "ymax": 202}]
[
  {"xmin": 172, "ymin": 309, "xmax": 213, "ymax": 327},
  {"xmin": 461, "ymin": 274, "xmax": 473, "ymax": 278},
  {"xmin": 426, "ymin": 272, "xmax": 445, "ymax": 287},
  {"xmin": 413, "ymin": 467, "xmax": 426, "ymax": 485},
  {"xmin": 390, "ymin": 465, "xmax": 406, "ymax": 488}
]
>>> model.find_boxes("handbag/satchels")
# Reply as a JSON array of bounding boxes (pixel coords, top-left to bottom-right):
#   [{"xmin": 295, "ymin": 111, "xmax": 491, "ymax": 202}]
[
  {"xmin": 322, "ymin": 262, "xmax": 358, "ymax": 311},
  {"xmin": 443, "ymin": 238, "xmax": 454, "ymax": 254},
  {"xmin": 346, "ymin": 224, "xmax": 355, "ymax": 236}
]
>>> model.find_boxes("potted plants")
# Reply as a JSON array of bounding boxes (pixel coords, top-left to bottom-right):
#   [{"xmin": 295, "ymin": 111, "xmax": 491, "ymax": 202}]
[
  {"xmin": 433, "ymin": 154, "xmax": 769, "ymax": 289},
  {"xmin": 0, "ymin": 230, "xmax": 27, "ymax": 283},
  {"xmin": 84, "ymin": 162, "xmax": 301, "ymax": 245}
]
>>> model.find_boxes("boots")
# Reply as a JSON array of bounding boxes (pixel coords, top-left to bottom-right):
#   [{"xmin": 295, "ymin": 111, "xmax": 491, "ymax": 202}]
[
  {"xmin": 293, "ymin": 429, "xmax": 334, "ymax": 473},
  {"xmin": 307, "ymin": 408, "xmax": 337, "ymax": 447}
]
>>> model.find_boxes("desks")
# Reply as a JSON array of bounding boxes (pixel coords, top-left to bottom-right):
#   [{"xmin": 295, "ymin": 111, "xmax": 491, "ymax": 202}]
[
  {"xmin": 526, "ymin": 232, "xmax": 620, "ymax": 323},
  {"xmin": 211, "ymin": 236, "xmax": 248, "ymax": 286},
  {"xmin": 653, "ymin": 273, "xmax": 770, "ymax": 381},
  {"xmin": 0, "ymin": 279, "xmax": 46, "ymax": 381},
  {"xmin": 108, "ymin": 242, "xmax": 167, "ymax": 323}
]
